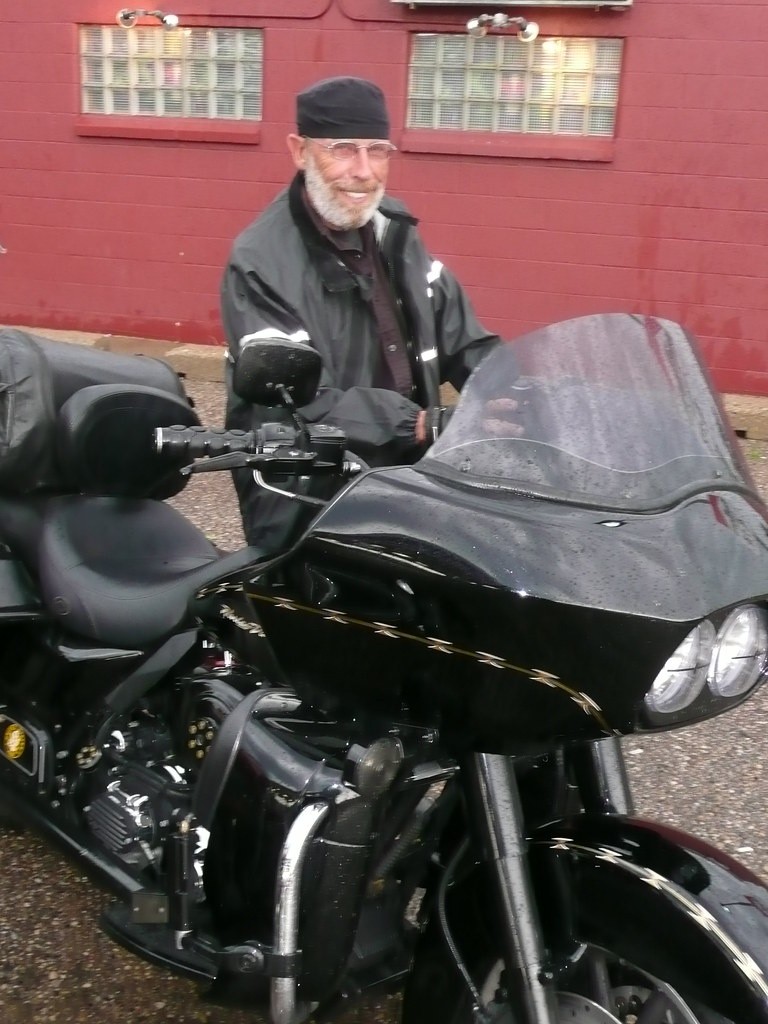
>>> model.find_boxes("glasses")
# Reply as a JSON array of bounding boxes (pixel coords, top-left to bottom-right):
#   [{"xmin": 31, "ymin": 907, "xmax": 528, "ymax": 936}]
[{"xmin": 302, "ymin": 134, "xmax": 397, "ymax": 162}]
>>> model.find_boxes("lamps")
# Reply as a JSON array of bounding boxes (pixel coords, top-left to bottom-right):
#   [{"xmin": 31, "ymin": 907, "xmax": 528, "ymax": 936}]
[
  {"xmin": 468, "ymin": 13, "xmax": 541, "ymax": 42},
  {"xmin": 116, "ymin": 8, "xmax": 179, "ymax": 31}
]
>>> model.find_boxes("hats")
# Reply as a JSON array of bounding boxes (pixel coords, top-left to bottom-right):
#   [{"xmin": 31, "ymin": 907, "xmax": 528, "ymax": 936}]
[{"xmin": 296, "ymin": 75, "xmax": 390, "ymax": 140}]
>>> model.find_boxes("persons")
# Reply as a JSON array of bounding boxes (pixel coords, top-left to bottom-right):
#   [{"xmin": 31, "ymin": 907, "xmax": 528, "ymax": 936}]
[{"xmin": 220, "ymin": 77, "xmax": 558, "ymax": 548}]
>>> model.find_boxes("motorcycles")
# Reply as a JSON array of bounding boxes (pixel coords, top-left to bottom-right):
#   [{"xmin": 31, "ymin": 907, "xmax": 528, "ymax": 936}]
[{"xmin": 1, "ymin": 307, "xmax": 767, "ymax": 1024}]
